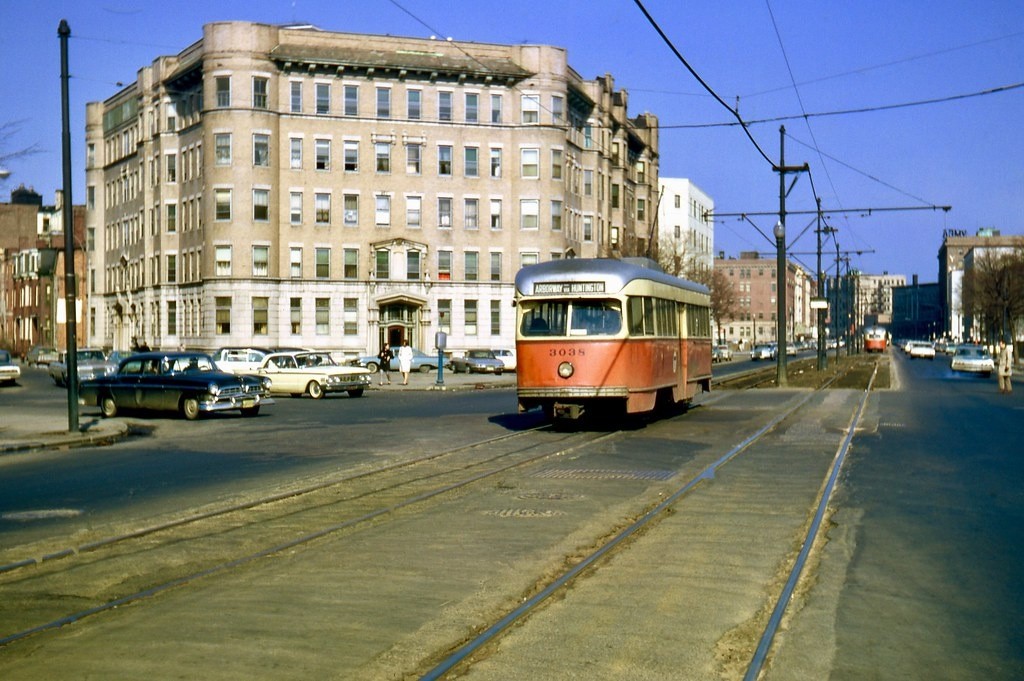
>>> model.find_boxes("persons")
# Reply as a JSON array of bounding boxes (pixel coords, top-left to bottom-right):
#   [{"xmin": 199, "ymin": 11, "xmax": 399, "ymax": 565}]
[
  {"xmin": 398, "ymin": 340, "xmax": 414, "ymax": 385},
  {"xmin": 150, "ymin": 360, "xmax": 159, "ymax": 375},
  {"xmin": 994, "ymin": 340, "xmax": 1013, "ymax": 394},
  {"xmin": 139, "ymin": 341, "xmax": 151, "ymax": 352},
  {"xmin": 284, "ymin": 359, "xmax": 295, "ymax": 368},
  {"xmin": 305, "ymin": 358, "xmax": 316, "ymax": 366},
  {"xmin": 377, "ymin": 343, "xmax": 394, "ymax": 386},
  {"xmin": 133, "ymin": 343, "xmax": 139, "ymax": 352}
]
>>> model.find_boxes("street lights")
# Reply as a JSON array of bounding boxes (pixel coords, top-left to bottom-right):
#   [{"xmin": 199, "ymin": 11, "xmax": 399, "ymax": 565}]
[
  {"xmin": 821, "ymin": 270, "xmax": 828, "ymax": 368},
  {"xmin": 773, "ymin": 220, "xmax": 788, "ymax": 386}
]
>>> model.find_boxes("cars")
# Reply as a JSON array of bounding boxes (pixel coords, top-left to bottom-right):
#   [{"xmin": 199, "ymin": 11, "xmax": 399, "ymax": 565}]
[
  {"xmin": 234, "ymin": 351, "xmax": 372, "ymax": 399},
  {"xmin": 26, "ymin": 345, "xmax": 60, "ymax": 366},
  {"xmin": 935, "ymin": 343, "xmax": 956, "ymax": 355},
  {"xmin": 775, "ymin": 341, "xmax": 797, "ymax": 357},
  {"xmin": 798, "ymin": 340, "xmax": 845, "ymax": 351},
  {"xmin": 450, "ymin": 348, "xmax": 516, "ymax": 377},
  {"xmin": 950, "ymin": 343, "xmax": 994, "ymax": 377},
  {"xmin": 749, "ymin": 343, "xmax": 776, "ymax": 361},
  {"xmin": 712, "ymin": 345, "xmax": 733, "ymax": 362},
  {"xmin": 47, "ymin": 348, "xmax": 117, "ymax": 387},
  {"xmin": 165, "ymin": 347, "xmax": 310, "ymax": 374},
  {"xmin": 350, "ymin": 346, "xmax": 449, "ymax": 373},
  {"xmin": 896, "ymin": 339, "xmax": 935, "ymax": 360},
  {"xmin": 0, "ymin": 349, "xmax": 21, "ymax": 383},
  {"xmin": 78, "ymin": 351, "xmax": 272, "ymax": 421}
]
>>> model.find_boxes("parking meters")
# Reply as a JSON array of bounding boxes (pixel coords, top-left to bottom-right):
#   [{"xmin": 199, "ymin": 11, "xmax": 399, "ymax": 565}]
[{"xmin": 435, "ymin": 332, "xmax": 447, "ymax": 385}]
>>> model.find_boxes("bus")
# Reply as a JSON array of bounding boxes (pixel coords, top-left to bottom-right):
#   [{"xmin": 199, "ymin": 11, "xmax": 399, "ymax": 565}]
[
  {"xmin": 863, "ymin": 326, "xmax": 886, "ymax": 353},
  {"xmin": 512, "ymin": 256, "xmax": 713, "ymax": 418}
]
